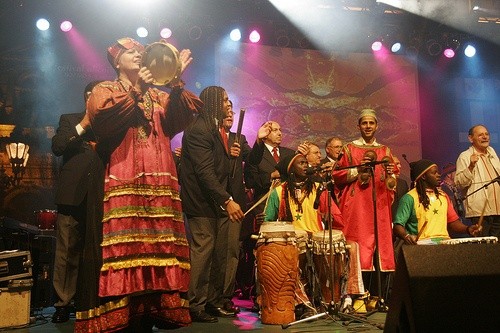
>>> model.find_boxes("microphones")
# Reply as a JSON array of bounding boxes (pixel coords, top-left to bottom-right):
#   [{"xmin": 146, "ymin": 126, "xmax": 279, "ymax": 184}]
[
  {"xmin": 307, "ymin": 166, "xmax": 330, "ymax": 176},
  {"xmin": 453, "ymin": 188, "xmax": 466, "ymax": 217},
  {"xmin": 314, "ymin": 184, "xmax": 323, "ymax": 210}
]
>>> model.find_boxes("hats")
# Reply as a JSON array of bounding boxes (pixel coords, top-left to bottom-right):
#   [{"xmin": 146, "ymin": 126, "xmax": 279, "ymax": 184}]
[
  {"xmin": 274, "ymin": 153, "xmax": 303, "ymax": 182},
  {"xmin": 357, "ymin": 108, "xmax": 377, "ymax": 124},
  {"xmin": 105, "ymin": 37, "xmax": 145, "ymax": 67},
  {"xmin": 409, "ymin": 159, "xmax": 437, "ymax": 182}
]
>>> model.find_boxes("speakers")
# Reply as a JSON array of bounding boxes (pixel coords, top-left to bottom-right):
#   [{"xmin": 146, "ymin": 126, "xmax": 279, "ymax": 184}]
[
  {"xmin": 383, "ymin": 244, "xmax": 500, "ymax": 333},
  {"xmin": 30, "ymin": 234, "xmax": 58, "ymax": 309}
]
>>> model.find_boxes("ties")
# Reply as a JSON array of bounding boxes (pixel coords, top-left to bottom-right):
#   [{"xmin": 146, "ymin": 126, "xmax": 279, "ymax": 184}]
[
  {"xmin": 220, "ymin": 127, "xmax": 229, "ymax": 154},
  {"xmin": 272, "ymin": 148, "xmax": 280, "ymax": 165}
]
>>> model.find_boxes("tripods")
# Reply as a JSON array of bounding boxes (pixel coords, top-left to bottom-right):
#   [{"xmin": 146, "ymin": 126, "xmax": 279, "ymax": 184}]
[{"xmin": 282, "ymin": 154, "xmax": 389, "ymax": 328}]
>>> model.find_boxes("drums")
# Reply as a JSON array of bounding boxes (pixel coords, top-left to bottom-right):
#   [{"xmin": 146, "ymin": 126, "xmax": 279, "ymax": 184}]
[
  {"xmin": 308, "ymin": 229, "xmax": 349, "ymax": 319},
  {"xmin": 138, "ymin": 40, "xmax": 182, "ymax": 87},
  {"xmin": 438, "ymin": 236, "xmax": 498, "ymax": 244},
  {"xmin": 31, "ymin": 208, "xmax": 59, "ymax": 239},
  {"xmin": 255, "ymin": 221, "xmax": 300, "ymax": 325}
]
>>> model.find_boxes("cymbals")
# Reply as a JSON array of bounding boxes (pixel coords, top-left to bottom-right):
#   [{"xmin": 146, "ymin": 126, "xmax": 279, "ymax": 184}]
[{"xmin": 137, "ymin": 39, "xmax": 184, "ymax": 88}]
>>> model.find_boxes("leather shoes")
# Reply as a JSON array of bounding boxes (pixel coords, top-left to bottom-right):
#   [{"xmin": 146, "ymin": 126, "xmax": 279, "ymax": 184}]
[
  {"xmin": 207, "ymin": 307, "xmax": 235, "ymax": 318},
  {"xmin": 224, "ymin": 303, "xmax": 241, "ymax": 313},
  {"xmin": 190, "ymin": 310, "xmax": 218, "ymax": 323}
]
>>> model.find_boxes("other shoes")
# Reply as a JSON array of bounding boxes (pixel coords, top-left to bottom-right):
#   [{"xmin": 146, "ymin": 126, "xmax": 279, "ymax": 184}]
[
  {"xmin": 368, "ymin": 296, "xmax": 378, "ymax": 309},
  {"xmin": 52, "ymin": 307, "xmax": 70, "ymax": 322},
  {"xmin": 352, "ymin": 300, "xmax": 367, "ymax": 314}
]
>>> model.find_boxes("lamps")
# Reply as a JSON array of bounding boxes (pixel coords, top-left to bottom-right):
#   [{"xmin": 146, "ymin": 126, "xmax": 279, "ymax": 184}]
[
  {"xmin": 189, "ymin": 18, "xmax": 460, "ymax": 58},
  {"xmin": 5, "ymin": 124, "xmax": 34, "ymax": 183}
]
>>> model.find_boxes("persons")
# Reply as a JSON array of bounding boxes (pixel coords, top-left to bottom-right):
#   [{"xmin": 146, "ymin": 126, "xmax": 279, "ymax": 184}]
[
  {"xmin": 75, "ymin": 38, "xmax": 196, "ymax": 333},
  {"xmin": 178, "ymin": 86, "xmax": 500, "ymax": 324},
  {"xmin": 50, "ymin": 79, "xmax": 109, "ymax": 323}
]
